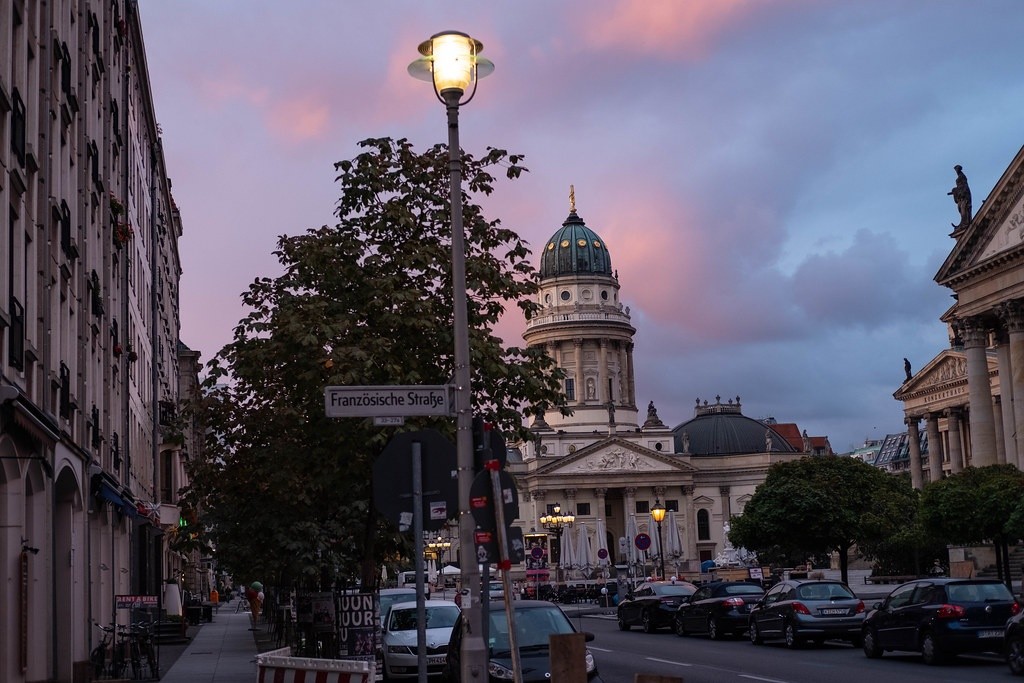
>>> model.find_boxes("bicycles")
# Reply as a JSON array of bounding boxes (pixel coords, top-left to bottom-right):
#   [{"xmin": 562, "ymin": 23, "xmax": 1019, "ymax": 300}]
[{"xmin": 89, "ymin": 619, "xmax": 163, "ymax": 683}]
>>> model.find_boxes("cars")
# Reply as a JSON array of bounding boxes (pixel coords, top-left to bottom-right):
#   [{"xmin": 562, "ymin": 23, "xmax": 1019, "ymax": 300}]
[
  {"xmin": 617, "ymin": 581, "xmax": 706, "ymax": 634},
  {"xmin": 860, "ymin": 579, "xmax": 1024, "ymax": 677},
  {"xmin": 747, "ymin": 580, "xmax": 865, "ymax": 650},
  {"xmin": 479, "ymin": 580, "xmax": 504, "ymax": 600},
  {"xmin": 375, "ymin": 588, "xmax": 467, "ymax": 683},
  {"xmin": 442, "ymin": 599, "xmax": 603, "ymax": 683},
  {"xmin": 670, "ymin": 582, "xmax": 770, "ymax": 640}
]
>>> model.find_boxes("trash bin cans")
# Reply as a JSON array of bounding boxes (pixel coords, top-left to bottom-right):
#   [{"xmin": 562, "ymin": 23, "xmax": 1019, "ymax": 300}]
[
  {"xmin": 188, "ymin": 606, "xmax": 200, "ymax": 626},
  {"xmin": 210, "ymin": 590, "xmax": 219, "ymax": 603},
  {"xmin": 203, "ymin": 607, "xmax": 213, "ymax": 623}
]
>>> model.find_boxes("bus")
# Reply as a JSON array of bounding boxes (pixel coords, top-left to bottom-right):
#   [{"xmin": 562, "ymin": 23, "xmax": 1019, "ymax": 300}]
[{"xmin": 397, "ymin": 571, "xmax": 430, "ymax": 600}]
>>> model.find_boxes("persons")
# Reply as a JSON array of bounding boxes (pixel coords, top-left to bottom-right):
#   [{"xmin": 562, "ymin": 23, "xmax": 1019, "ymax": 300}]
[
  {"xmin": 629, "ymin": 451, "xmax": 638, "ymax": 468},
  {"xmin": 715, "ymin": 551, "xmax": 757, "ymax": 567},
  {"xmin": 607, "ymin": 399, "xmax": 615, "ymax": 424},
  {"xmin": 824, "ymin": 436, "xmax": 831, "ymax": 456},
  {"xmin": 225, "ymin": 586, "xmax": 231, "ymax": 604},
  {"xmin": 597, "ymin": 573, "xmax": 606, "ymax": 585},
  {"xmin": 683, "ymin": 429, "xmax": 689, "ymax": 453},
  {"xmin": 647, "ymin": 400, "xmax": 657, "ymax": 416},
  {"xmin": 802, "ymin": 429, "xmax": 810, "ymax": 453},
  {"xmin": 588, "ymin": 381, "xmax": 595, "ymax": 400},
  {"xmin": 513, "ymin": 581, "xmax": 527, "ymax": 595},
  {"xmin": 948, "ymin": 165, "xmax": 973, "ymax": 229},
  {"xmin": 903, "ymin": 357, "xmax": 913, "ymax": 384},
  {"xmin": 598, "ymin": 455, "xmax": 616, "ymax": 468},
  {"xmin": 723, "ymin": 522, "xmax": 732, "ymax": 548},
  {"xmin": 765, "ymin": 428, "xmax": 772, "ymax": 451}
]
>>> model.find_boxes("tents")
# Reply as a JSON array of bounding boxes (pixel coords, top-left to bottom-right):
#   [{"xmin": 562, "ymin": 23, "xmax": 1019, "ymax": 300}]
[{"xmin": 436, "ymin": 563, "xmax": 497, "ymax": 586}]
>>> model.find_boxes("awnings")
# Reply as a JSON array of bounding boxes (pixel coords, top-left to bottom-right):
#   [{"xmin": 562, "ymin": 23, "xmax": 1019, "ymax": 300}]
[
  {"xmin": 12, "ymin": 399, "xmax": 60, "ymax": 450},
  {"xmin": 97, "ymin": 475, "xmax": 138, "ymax": 515}
]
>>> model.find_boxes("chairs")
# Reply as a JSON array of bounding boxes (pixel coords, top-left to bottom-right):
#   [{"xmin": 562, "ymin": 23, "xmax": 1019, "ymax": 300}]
[{"xmin": 802, "ymin": 585, "xmax": 843, "ymax": 597}]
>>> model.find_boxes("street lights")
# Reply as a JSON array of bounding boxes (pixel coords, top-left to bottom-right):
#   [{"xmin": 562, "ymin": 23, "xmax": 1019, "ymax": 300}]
[
  {"xmin": 650, "ymin": 495, "xmax": 666, "ymax": 581},
  {"xmin": 408, "ymin": 26, "xmax": 495, "ymax": 683},
  {"xmin": 429, "ymin": 534, "xmax": 451, "ymax": 589},
  {"xmin": 539, "ymin": 502, "xmax": 575, "ymax": 568}
]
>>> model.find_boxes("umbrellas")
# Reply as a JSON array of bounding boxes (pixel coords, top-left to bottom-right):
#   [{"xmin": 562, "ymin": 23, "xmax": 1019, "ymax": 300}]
[
  {"xmin": 596, "ymin": 518, "xmax": 613, "ymax": 608},
  {"xmin": 382, "ymin": 565, "xmax": 387, "ymax": 587},
  {"xmin": 559, "ymin": 523, "xmax": 576, "ymax": 588},
  {"xmin": 667, "ymin": 510, "xmax": 683, "ymax": 581},
  {"xmin": 647, "ymin": 513, "xmax": 662, "ymax": 580},
  {"xmin": 428, "ymin": 560, "xmax": 437, "ymax": 586},
  {"xmin": 626, "ymin": 513, "xmax": 646, "ymax": 588},
  {"xmin": 577, "ymin": 522, "xmax": 595, "ymax": 588}
]
